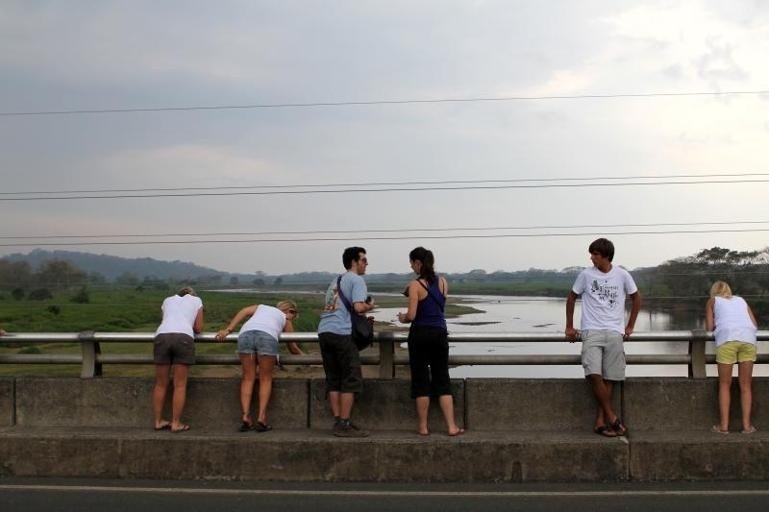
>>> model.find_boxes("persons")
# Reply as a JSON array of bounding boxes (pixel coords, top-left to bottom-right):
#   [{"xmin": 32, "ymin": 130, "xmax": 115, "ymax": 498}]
[
  {"xmin": 216, "ymin": 301, "xmax": 303, "ymax": 432},
  {"xmin": 566, "ymin": 238, "xmax": 640, "ymax": 437},
  {"xmin": 317, "ymin": 248, "xmax": 375, "ymax": 437},
  {"xmin": 707, "ymin": 281, "xmax": 758, "ymax": 433},
  {"xmin": 153, "ymin": 287, "xmax": 204, "ymax": 432},
  {"xmin": 397, "ymin": 247, "xmax": 464, "ymax": 435}
]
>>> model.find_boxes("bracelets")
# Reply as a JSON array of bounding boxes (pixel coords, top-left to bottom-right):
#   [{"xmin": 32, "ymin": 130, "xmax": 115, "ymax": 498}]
[{"xmin": 226, "ymin": 328, "xmax": 232, "ymax": 333}]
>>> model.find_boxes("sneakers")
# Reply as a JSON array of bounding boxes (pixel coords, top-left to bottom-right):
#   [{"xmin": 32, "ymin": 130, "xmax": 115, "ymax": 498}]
[{"xmin": 330, "ymin": 425, "xmax": 372, "ymax": 440}]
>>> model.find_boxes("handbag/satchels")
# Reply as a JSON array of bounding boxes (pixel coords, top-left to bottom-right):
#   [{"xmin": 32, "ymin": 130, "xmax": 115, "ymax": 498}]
[{"xmin": 349, "ymin": 314, "xmax": 373, "ymax": 353}]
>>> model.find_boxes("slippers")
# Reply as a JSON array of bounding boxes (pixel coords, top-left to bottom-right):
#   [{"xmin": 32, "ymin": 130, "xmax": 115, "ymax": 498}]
[
  {"xmin": 706, "ymin": 424, "xmax": 762, "ymax": 436},
  {"xmin": 414, "ymin": 427, "xmax": 469, "ymax": 439},
  {"xmin": 238, "ymin": 420, "xmax": 273, "ymax": 435},
  {"xmin": 593, "ymin": 418, "xmax": 627, "ymax": 439},
  {"xmin": 153, "ymin": 422, "xmax": 192, "ymax": 438}
]
